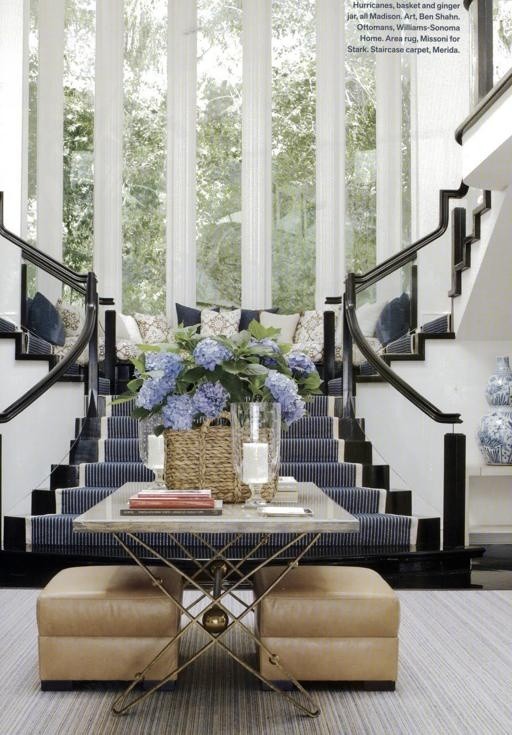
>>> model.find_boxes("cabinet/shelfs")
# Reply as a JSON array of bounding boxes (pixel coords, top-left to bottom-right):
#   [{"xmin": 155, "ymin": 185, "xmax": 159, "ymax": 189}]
[{"xmin": 465, "ymin": 466, "xmax": 512, "ymax": 572}]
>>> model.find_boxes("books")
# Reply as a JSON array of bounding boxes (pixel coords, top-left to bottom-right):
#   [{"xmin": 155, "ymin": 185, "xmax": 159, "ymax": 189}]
[
  {"xmin": 120, "ymin": 500, "xmax": 224, "ymax": 516},
  {"xmin": 138, "ymin": 489, "xmax": 211, "ymax": 498},
  {"xmin": 250, "ymin": 476, "xmax": 298, "ymax": 492},
  {"xmin": 127, "ymin": 493, "xmax": 214, "ymax": 509},
  {"xmin": 257, "ymin": 506, "xmax": 313, "ymax": 518}
]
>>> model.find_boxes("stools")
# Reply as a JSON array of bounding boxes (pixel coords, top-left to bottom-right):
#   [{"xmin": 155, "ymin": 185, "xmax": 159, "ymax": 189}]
[
  {"xmin": 34, "ymin": 564, "xmax": 182, "ymax": 694},
  {"xmin": 252, "ymin": 565, "xmax": 400, "ymax": 693}
]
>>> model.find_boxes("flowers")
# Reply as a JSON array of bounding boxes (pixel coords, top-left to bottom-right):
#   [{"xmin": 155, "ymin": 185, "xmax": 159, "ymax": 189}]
[{"xmin": 104, "ymin": 318, "xmax": 326, "ymax": 437}]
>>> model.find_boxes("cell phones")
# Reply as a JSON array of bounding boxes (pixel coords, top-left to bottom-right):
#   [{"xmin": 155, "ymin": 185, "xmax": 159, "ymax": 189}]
[{"xmin": 257, "ymin": 506, "xmax": 314, "ymax": 516}]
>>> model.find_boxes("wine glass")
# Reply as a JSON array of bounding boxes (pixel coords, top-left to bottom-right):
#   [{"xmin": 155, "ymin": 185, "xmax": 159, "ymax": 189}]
[
  {"xmin": 230, "ymin": 402, "xmax": 282, "ymax": 509},
  {"xmin": 137, "ymin": 411, "xmax": 168, "ymax": 490}
]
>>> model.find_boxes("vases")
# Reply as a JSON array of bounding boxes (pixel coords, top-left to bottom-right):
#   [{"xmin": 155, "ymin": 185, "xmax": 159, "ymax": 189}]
[
  {"xmin": 475, "ymin": 355, "xmax": 511, "ymax": 466},
  {"xmin": 160, "ymin": 412, "xmax": 281, "ymax": 505}
]
